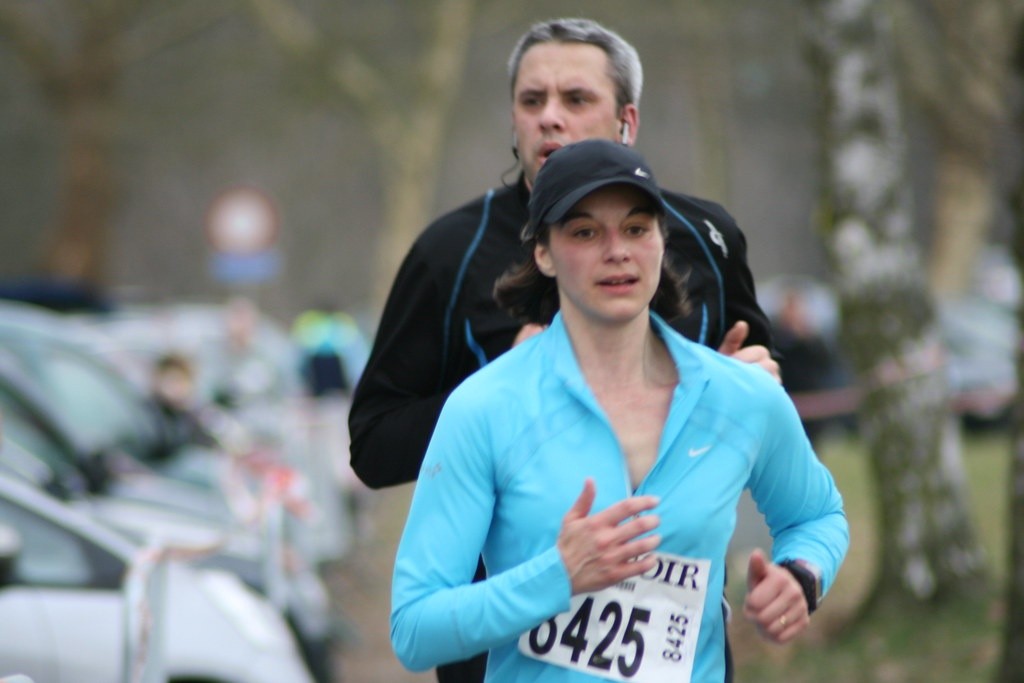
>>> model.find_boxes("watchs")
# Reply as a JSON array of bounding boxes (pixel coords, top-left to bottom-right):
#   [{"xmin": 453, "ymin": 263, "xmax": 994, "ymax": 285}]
[{"xmin": 785, "ymin": 558, "xmax": 822, "ymax": 614}]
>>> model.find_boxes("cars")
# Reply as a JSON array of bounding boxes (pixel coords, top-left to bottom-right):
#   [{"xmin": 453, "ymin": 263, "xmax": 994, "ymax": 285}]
[{"xmin": 0, "ymin": 267, "xmax": 375, "ymax": 683}]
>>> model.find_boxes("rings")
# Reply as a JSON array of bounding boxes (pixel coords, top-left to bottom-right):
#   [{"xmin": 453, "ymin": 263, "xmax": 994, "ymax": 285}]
[{"xmin": 780, "ymin": 615, "xmax": 786, "ymax": 628}]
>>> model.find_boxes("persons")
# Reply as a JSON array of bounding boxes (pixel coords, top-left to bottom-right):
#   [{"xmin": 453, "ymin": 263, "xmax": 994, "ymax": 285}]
[
  {"xmin": 348, "ymin": 18, "xmax": 781, "ymax": 683},
  {"xmin": 391, "ymin": 138, "xmax": 850, "ymax": 683}
]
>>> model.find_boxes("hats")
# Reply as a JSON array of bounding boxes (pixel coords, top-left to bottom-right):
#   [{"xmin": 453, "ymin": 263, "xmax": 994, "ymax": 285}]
[{"xmin": 527, "ymin": 137, "xmax": 667, "ymax": 237}]
